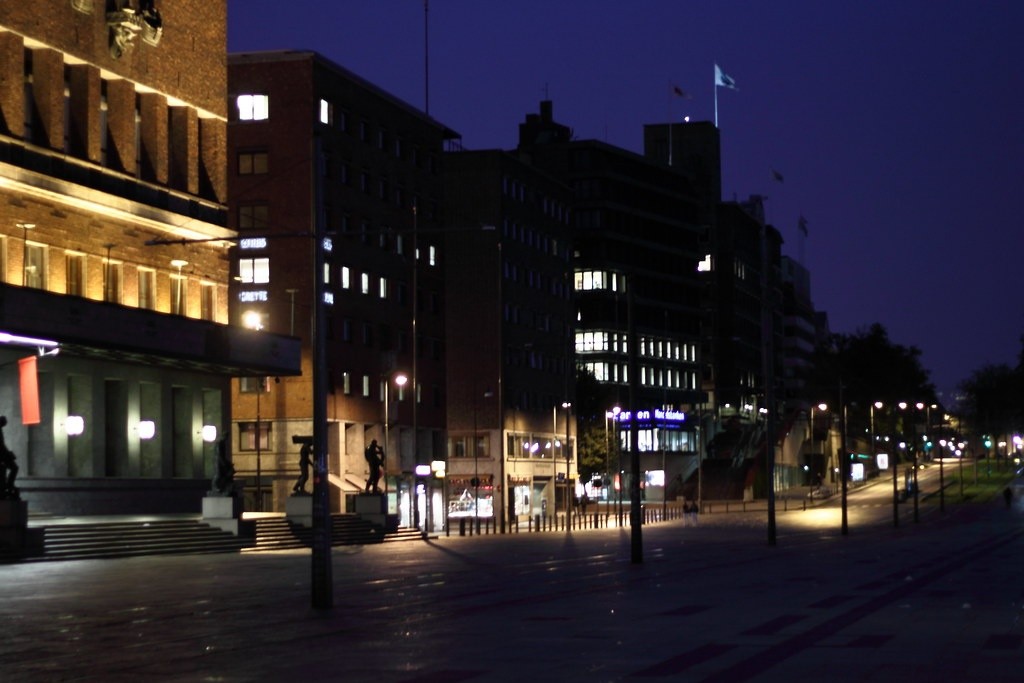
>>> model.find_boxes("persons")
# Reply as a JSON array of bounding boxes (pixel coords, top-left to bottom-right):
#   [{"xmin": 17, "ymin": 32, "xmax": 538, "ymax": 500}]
[
  {"xmin": 572, "ymin": 494, "xmax": 579, "ymax": 515},
  {"xmin": 460, "ymin": 489, "xmax": 473, "ymax": 501},
  {"xmin": 0, "ymin": 416, "xmax": 19, "ymax": 491},
  {"xmin": 1003, "ymin": 487, "xmax": 1013, "ymax": 508},
  {"xmin": 212, "ymin": 431, "xmax": 234, "ymax": 491},
  {"xmin": 365, "ymin": 439, "xmax": 384, "ymax": 492},
  {"xmin": 682, "ymin": 501, "xmax": 698, "ymax": 526},
  {"xmin": 293, "ymin": 436, "xmax": 313, "ymax": 492},
  {"xmin": 580, "ymin": 493, "xmax": 589, "ymax": 514},
  {"xmin": 816, "ymin": 473, "xmax": 821, "ymax": 489}
]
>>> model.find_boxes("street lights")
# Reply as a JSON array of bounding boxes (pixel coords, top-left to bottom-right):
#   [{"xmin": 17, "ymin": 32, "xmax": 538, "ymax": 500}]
[
  {"xmin": 890, "ymin": 402, "xmax": 908, "ymax": 527},
  {"xmin": 870, "ymin": 401, "xmax": 883, "ymax": 478},
  {"xmin": 562, "ymin": 401, "xmax": 572, "ymax": 532},
  {"xmin": 911, "ymin": 402, "xmax": 924, "ymax": 522},
  {"xmin": 927, "ymin": 404, "xmax": 963, "ymax": 513},
  {"xmin": 611, "ymin": 405, "xmax": 621, "ymax": 501},
  {"xmin": 810, "ymin": 403, "xmax": 828, "ymax": 503},
  {"xmin": 473, "ymin": 389, "xmax": 495, "ymax": 536},
  {"xmin": 384, "ymin": 370, "xmax": 407, "ymax": 513}
]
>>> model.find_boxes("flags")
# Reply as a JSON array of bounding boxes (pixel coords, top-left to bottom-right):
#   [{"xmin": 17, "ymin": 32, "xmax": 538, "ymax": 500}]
[
  {"xmin": 800, "ymin": 217, "xmax": 808, "ymax": 234},
  {"xmin": 770, "ymin": 169, "xmax": 783, "ymax": 182},
  {"xmin": 715, "ymin": 64, "xmax": 735, "ymax": 88},
  {"xmin": 671, "ymin": 82, "xmax": 691, "ymax": 100}
]
